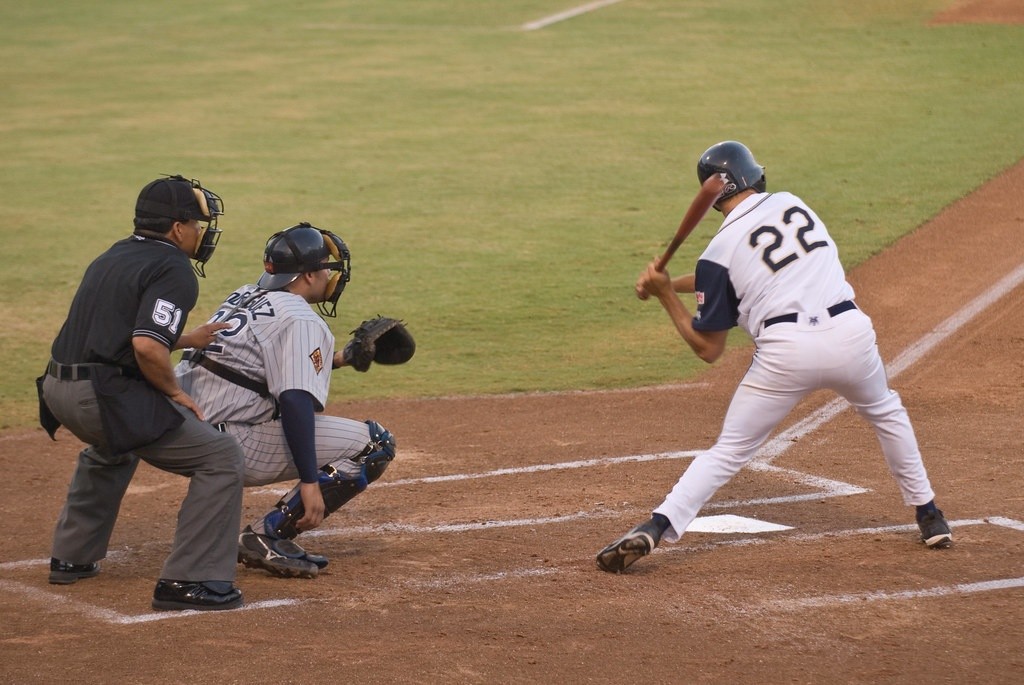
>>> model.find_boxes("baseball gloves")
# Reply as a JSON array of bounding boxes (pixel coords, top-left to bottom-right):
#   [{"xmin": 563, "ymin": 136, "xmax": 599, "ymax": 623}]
[{"xmin": 342, "ymin": 316, "xmax": 417, "ymax": 374}]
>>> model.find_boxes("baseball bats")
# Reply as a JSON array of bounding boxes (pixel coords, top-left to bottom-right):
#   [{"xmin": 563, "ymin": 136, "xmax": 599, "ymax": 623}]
[{"xmin": 638, "ymin": 174, "xmax": 723, "ymax": 301}]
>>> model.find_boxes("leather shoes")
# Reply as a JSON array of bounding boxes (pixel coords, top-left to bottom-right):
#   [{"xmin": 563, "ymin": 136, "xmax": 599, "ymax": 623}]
[
  {"xmin": 151, "ymin": 578, "xmax": 244, "ymax": 610},
  {"xmin": 49, "ymin": 557, "xmax": 99, "ymax": 584}
]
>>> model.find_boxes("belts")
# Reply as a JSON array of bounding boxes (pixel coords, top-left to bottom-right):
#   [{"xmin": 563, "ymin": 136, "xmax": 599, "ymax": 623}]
[
  {"xmin": 47, "ymin": 360, "xmax": 93, "ymax": 380},
  {"xmin": 214, "ymin": 423, "xmax": 225, "ymax": 431},
  {"xmin": 764, "ymin": 299, "xmax": 857, "ymax": 331}
]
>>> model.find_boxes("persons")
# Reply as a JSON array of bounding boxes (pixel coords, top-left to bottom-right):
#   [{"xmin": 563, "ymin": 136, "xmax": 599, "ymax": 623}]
[
  {"xmin": 34, "ymin": 173, "xmax": 242, "ymax": 609},
  {"xmin": 597, "ymin": 141, "xmax": 953, "ymax": 572},
  {"xmin": 174, "ymin": 222, "xmax": 417, "ymax": 579}
]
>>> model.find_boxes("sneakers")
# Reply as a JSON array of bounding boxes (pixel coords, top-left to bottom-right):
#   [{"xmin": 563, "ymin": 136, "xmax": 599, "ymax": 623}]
[
  {"xmin": 915, "ymin": 508, "xmax": 952, "ymax": 548},
  {"xmin": 238, "ymin": 524, "xmax": 329, "ymax": 578},
  {"xmin": 596, "ymin": 526, "xmax": 660, "ymax": 574}
]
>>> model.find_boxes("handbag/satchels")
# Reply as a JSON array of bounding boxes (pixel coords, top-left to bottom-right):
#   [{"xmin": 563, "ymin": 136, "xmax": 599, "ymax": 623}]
[
  {"xmin": 35, "ymin": 379, "xmax": 63, "ymax": 440},
  {"xmin": 90, "ymin": 364, "xmax": 186, "ymax": 456}
]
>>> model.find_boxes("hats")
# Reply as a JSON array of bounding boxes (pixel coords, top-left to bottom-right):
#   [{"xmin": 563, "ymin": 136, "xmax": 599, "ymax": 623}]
[{"xmin": 135, "ymin": 174, "xmax": 219, "ymax": 221}]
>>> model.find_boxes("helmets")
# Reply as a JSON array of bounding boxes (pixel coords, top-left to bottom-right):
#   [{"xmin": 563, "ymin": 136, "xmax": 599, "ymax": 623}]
[
  {"xmin": 697, "ymin": 140, "xmax": 765, "ymax": 212},
  {"xmin": 258, "ymin": 228, "xmax": 327, "ymax": 289}
]
